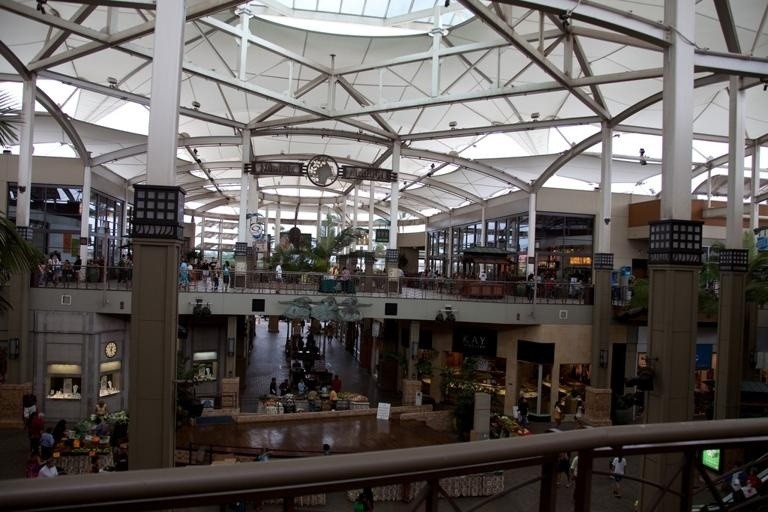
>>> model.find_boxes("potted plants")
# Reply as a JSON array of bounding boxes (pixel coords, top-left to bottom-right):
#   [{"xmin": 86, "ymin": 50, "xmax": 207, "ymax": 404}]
[{"xmin": 175, "ymin": 354, "xmax": 213, "ymax": 397}]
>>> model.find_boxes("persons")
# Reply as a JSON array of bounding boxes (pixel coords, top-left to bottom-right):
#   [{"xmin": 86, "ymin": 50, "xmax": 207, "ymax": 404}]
[
  {"xmin": 276, "ymin": 264, "xmax": 282, "ymax": 283},
  {"xmin": 419, "ymin": 266, "xmax": 592, "ymax": 304},
  {"xmin": 748, "ymin": 467, "xmax": 763, "ymax": 494},
  {"xmin": 36, "ymin": 401, "xmax": 128, "ymax": 477},
  {"xmin": 518, "ymin": 395, "xmax": 585, "ymax": 430},
  {"xmin": 289, "ymin": 335, "xmax": 318, "ymax": 360},
  {"xmin": 178, "ymin": 254, "xmax": 235, "ymax": 292},
  {"xmin": 270, "ymin": 369, "xmax": 342, "ymax": 414},
  {"xmin": 325, "ymin": 323, "xmax": 335, "ymax": 344},
  {"xmin": 329, "ymin": 265, "xmax": 362, "ymax": 292},
  {"xmin": 730, "ymin": 471, "xmax": 748, "ymax": 503},
  {"xmin": 301, "ymin": 319, "xmax": 305, "ymax": 332},
  {"xmin": 398, "ymin": 269, "xmax": 404, "ymax": 294},
  {"xmin": 31, "ymin": 253, "xmax": 133, "ymax": 287}
]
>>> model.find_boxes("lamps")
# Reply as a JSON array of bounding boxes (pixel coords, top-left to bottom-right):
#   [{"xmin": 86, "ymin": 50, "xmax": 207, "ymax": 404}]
[
  {"xmin": 436, "ymin": 307, "xmax": 456, "ymax": 324},
  {"xmin": 187, "ymin": 300, "xmax": 214, "ymax": 318},
  {"xmin": 412, "ymin": 343, "xmax": 419, "ymax": 360},
  {"xmin": 599, "ymin": 350, "xmax": 608, "ymax": 368},
  {"xmin": 8, "ymin": 338, "xmax": 19, "ymax": 360},
  {"xmin": 228, "ymin": 338, "xmax": 235, "ymax": 356}
]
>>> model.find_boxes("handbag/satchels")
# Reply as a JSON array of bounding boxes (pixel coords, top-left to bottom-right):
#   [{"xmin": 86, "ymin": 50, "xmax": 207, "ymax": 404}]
[{"xmin": 224, "ymin": 269, "xmax": 229, "ymax": 276}]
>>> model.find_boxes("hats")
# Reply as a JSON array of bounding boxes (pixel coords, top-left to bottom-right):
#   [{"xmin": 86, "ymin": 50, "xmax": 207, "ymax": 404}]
[{"xmin": 47, "ymin": 459, "xmax": 56, "ymax": 463}]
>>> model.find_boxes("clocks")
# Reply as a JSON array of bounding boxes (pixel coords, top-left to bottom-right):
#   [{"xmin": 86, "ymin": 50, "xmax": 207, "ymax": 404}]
[{"xmin": 105, "ymin": 341, "xmax": 117, "ymax": 358}]
